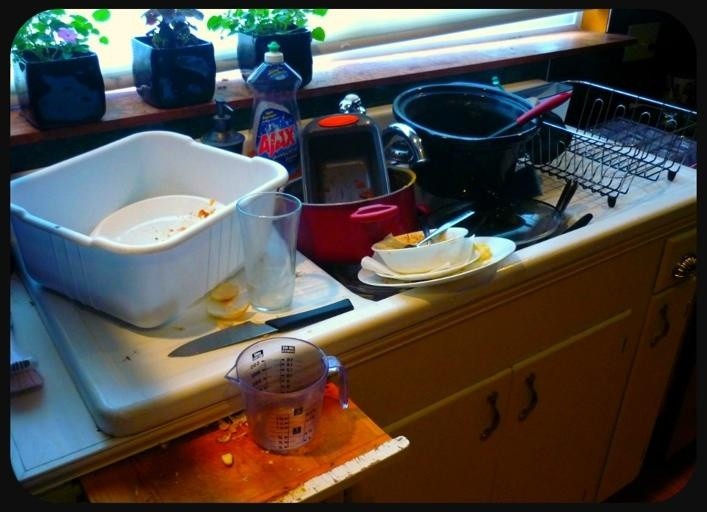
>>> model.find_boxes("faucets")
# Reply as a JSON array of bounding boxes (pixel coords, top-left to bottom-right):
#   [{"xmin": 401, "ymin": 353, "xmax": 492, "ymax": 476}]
[{"xmin": 330, "ymin": 93, "xmax": 427, "ymax": 170}]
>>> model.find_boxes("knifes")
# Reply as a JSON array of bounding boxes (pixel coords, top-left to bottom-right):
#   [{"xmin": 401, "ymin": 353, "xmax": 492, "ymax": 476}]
[{"xmin": 165, "ymin": 298, "xmax": 354, "ymax": 357}]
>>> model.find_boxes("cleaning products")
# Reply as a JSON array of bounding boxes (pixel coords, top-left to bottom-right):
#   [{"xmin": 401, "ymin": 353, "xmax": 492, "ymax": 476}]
[
  {"xmin": 247, "ymin": 41, "xmax": 301, "ymax": 169},
  {"xmin": 196, "ymin": 94, "xmax": 245, "ymax": 163}
]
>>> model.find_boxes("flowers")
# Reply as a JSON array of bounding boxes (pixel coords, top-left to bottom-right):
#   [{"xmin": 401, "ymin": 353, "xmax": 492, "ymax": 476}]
[{"xmin": 10, "ymin": 10, "xmax": 112, "ymax": 63}]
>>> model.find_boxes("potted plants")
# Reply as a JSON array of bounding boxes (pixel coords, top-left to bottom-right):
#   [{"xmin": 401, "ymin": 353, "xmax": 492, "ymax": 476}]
[
  {"xmin": 207, "ymin": 8, "xmax": 327, "ymax": 92},
  {"xmin": 129, "ymin": 8, "xmax": 217, "ymax": 110}
]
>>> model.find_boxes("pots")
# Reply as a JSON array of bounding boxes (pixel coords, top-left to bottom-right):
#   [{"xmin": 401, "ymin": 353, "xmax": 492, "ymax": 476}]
[
  {"xmin": 278, "ymin": 162, "xmax": 417, "ymax": 262},
  {"xmin": 392, "ymin": 80, "xmax": 542, "ymax": 201}
]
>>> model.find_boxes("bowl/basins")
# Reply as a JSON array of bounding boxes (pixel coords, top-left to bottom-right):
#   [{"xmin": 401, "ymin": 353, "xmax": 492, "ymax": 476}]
[{"xmin": 371, "ymin": 226, "xmax": 469, "ymax": 274}]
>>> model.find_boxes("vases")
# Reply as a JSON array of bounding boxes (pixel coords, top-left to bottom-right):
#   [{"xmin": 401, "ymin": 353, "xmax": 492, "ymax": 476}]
[{"xmin": 10, "ymin": 47, "xmax": 106, "ymax": 130}]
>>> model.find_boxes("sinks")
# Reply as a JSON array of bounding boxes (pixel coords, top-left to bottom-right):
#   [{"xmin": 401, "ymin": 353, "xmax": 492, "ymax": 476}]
[{"xmin": 265, "ymin": 177, "xmax": 587, "ymax": 306}]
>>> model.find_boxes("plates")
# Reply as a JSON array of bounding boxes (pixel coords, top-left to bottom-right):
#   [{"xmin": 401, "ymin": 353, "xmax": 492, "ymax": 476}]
[
  {"xmin": 357, "ymin": 235, "xmax": 517, "ymax": 288},
  {"xmin": 377, "ymin": 250, "xmax": 480, "ymax": 278},
  {"xmin": 91, "ymin": 194, "xmax": 226, "ymax": 245}
]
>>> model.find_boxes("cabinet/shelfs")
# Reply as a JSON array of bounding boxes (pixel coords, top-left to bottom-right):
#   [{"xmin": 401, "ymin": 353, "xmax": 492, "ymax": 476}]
[
  {"xmin": 597, "ymin": 229, "xmax": 697, "ymax": 503},
  {"xmin": 344, "ymin": 242, "xmax": 664, "ymax": 503}
]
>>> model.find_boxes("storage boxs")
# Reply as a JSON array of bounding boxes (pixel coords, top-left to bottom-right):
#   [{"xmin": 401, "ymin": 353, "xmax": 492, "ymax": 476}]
[{"xmin": 10, "ymin": 129, "xmax": 289, "ymax": 331}]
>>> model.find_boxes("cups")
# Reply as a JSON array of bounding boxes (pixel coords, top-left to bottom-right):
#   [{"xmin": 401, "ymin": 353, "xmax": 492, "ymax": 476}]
[
  {"xmin": 224, "ymin": 336, "xmax": 350, "ymax": 456},
  {"xmin": 237, "ymin": 191, "xmax": 302, "ymax": 313}
]
